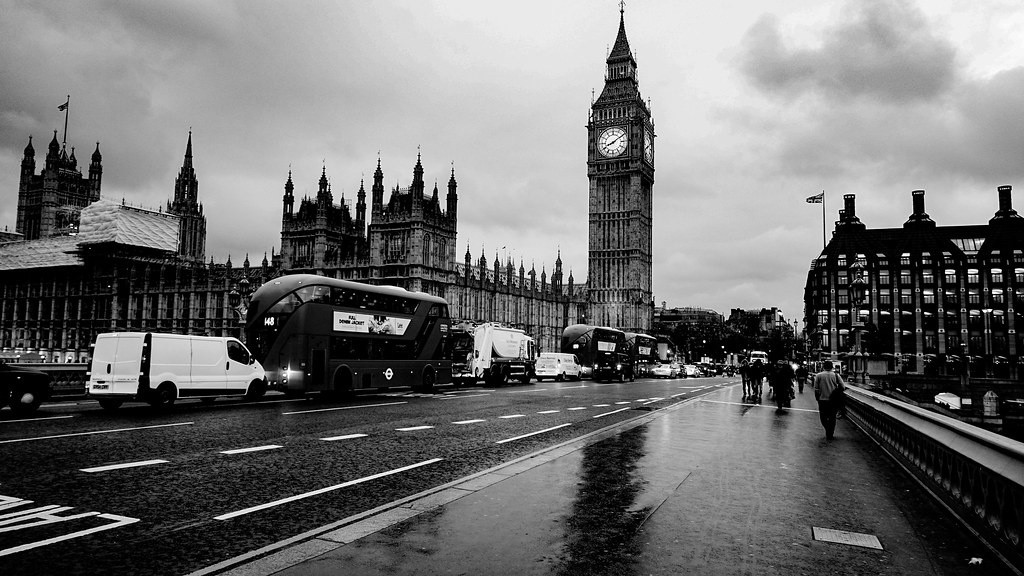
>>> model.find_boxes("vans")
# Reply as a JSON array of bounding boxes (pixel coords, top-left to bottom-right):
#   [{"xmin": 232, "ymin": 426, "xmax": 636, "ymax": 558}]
[
  {"xmin": 535, "ymin": 353, "xmax": 583, "ymax": 380},
  {"xmin": 87, "ymin": 334, "xmax": 270, "ymax": 410}
]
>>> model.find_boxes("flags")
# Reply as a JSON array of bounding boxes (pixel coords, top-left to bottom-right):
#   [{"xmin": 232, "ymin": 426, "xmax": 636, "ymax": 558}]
[
  {"xmin": 806, "ymin": 193, "xmax": 822, "ymax": 204},
  {"xmin": 57, "ymin": 102, "xmax": 67, "ymax": 112}
]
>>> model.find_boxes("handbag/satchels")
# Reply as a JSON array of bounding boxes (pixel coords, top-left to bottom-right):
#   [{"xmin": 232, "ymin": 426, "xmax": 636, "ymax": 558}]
[{"xmin": 781, "ymin": 394, "xmax": 793, "ymax": 408}]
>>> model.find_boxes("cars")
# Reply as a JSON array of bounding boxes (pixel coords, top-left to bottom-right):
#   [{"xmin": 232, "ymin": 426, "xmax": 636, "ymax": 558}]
[
  {"xmin": 652, "ymin": 361, "xmax": 739, "ymax": 379},
  {"xmin": 0, "ymin": 359, "xmax": 50, "ymax": 418}
]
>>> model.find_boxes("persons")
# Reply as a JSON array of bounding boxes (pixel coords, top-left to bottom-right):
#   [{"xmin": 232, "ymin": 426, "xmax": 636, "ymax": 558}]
[
  {"xmin": 723, "ymin": 357, "xmax": 808, "ymax": 411},
  {"xmin": 842, "ymin": 365, "xmax": 848, "ymax": 382},
  {"xmin": 815, "ymin": 360, "xmax": 845, "ymax": 439}
]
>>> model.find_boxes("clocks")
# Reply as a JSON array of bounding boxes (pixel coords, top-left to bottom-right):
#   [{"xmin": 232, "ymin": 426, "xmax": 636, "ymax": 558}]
[
  {"xmin": 644, "ymin": 127, "xmax": 653, "ymax": 166},
  {"xmin": 598, "ymin": 127, "xmax": 627, "ymax": 157}
]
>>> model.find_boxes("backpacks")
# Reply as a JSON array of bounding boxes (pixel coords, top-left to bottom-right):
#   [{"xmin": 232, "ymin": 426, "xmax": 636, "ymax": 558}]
[{"xmin": 830, "ymin": 386, "xmax": 847, "ymax": 407}]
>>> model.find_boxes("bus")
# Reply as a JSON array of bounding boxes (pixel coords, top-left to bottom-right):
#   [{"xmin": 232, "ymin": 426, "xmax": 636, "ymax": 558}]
[
  {"xmin": 563, "ymin": 324, "xmax": 636, "ymax": 383},
  {"xmin": 243, "ymin": 273, "xmax": 458, "ymax": 401},
  {"xmin": 622, "ymin": 332, "xmax": 660, "ymax": 378}
]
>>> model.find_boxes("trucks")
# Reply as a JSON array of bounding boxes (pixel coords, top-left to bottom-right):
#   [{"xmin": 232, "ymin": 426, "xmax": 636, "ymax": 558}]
[{"xmin": 448, "ymin": 320, "xmax": 541, "ymax": 388}]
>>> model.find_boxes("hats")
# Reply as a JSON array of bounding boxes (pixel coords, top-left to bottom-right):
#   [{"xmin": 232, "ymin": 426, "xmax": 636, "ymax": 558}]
[{"xmin": 777, "ymin": 360, "xmax": 784, "ymax": 364}]
